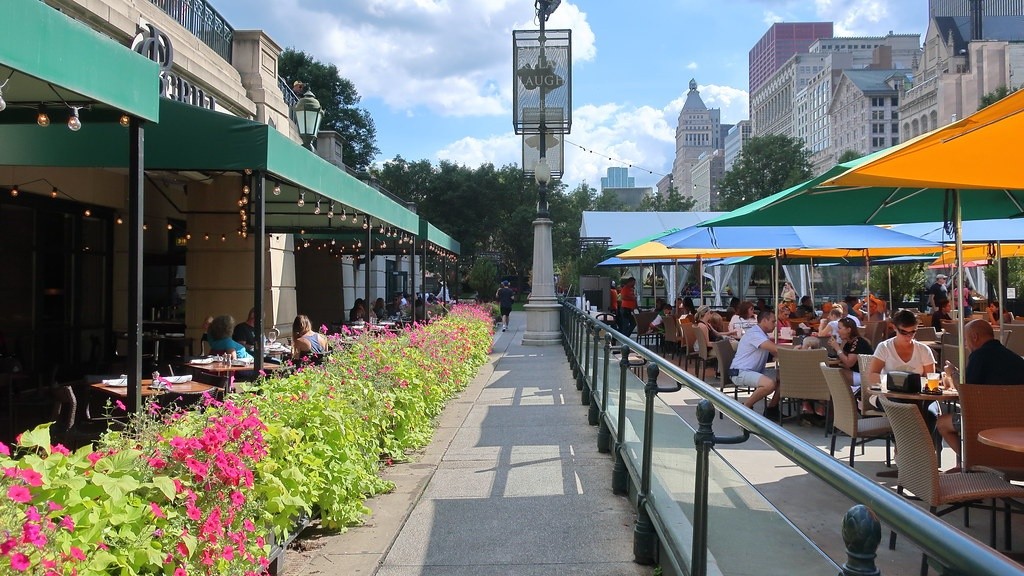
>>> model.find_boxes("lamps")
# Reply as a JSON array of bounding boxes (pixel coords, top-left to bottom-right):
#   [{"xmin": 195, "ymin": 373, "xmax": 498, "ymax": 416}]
[{"xmin": 292, "ymin": 87, "xmax": 326, "ymax": 154}]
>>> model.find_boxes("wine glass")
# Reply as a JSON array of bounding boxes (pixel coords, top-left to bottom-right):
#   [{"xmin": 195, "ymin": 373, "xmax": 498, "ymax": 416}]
[{"xmin": 268, "ymin": 332, "xmax": 277, "ymax": 345}]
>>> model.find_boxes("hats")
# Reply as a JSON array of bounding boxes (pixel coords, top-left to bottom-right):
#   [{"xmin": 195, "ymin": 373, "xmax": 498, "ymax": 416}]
[
  {"xmin": 936, "ymin": 274, "xmax": 947, "ymax": 278},
  {"xmin": 503, "ymin": 280, "xmax": 508, "ymax": 286},
  {"xmin": 697, "ymin": 305, "xmax": 708, "ymax": 315},
  {"xmin": 440, "ymin": 281, "xmax": 447, "ymax": 285}
]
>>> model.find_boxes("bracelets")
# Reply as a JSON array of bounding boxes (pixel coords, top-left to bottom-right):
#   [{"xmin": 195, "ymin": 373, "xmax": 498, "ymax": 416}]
[
  {"xmin": 836, "ymin": 350, "xmax": 843, "ymax": 356},
  {"xmin": 951, "ymin": 372, "xmax": 960, "ymax": 378}
]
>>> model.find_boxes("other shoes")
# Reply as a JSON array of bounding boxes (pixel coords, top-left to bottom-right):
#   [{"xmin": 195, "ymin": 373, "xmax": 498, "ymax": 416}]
[
  {"xmin": 502, "ymin": 327, "xmax": 505, "ymax": 332},
  {"xmin": 763, "ymin": 406, "xmax": 788, "ymax": 418},
  {"xmin": 506, "ymin": 326, "xmax": 509, "ymax": 331}
]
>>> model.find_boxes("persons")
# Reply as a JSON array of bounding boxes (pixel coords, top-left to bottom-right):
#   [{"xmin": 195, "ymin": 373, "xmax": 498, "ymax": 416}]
[
  {"xmin": 207, "ymin": 315, "xmax": 254, "ymax": 363},
  {"xmin": 349, "ymin": 293, "xmax": 441, "ymax": 324},
  {"xmin": 936, "ymin": 319, "xmax": 1024, "ymax": 472},
  {"xmin": 496, "ymin": 281, "xmax": 514, "ymax": 332},
  {"xmin": 292, "ymin": 315, "xmax": 328, "ymax": 360},
  {"xmin": 231, "ymin": 307, "xmax": 266, "ymax": 351},
  {"xmin": 610, "ymin": 273, "xmax": 1011, "ymax": 425},
  {"xmin": 868, "ymin": 310, "xmax": 940, "ymax": 468}
]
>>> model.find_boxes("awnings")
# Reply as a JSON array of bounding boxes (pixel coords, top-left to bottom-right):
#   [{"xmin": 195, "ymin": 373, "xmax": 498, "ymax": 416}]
[
  {"xmin": 294, "ymin": 219, "xmax": 461, "ymax": 315},
  {"xmin": 0, "ymin": 95, "xmax": 420, "ymax": 386},
  {"xmin": 0, "ymin": 0, "xmax": 162, "ymax": 438}
]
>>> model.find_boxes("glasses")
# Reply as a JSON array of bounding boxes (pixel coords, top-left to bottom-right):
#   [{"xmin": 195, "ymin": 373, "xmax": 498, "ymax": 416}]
[
  {"xmin": 897, "ymin": 327, "xmax": 916, "ymax": 335},
  {"xmin": 843, "ymin": 316, "xmax": 849, "ymax": 322}
]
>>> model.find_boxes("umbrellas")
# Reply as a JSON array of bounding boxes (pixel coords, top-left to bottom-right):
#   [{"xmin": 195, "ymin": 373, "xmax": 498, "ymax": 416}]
[
  {"xmin": 821, "ymin": 88, "xmax": 1024, "ymax": 189},
  {"xmin": 697, "ymin": 145, "xmax": 1024, "ymax": 475},
  {"xmin": 593, "ymin": 218, "xmax": 1024, "ymax": 372}
]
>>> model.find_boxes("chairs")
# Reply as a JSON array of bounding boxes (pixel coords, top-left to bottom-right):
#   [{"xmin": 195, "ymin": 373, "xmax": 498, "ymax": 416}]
[
  {"xmin": 40, "ymin": 375, "xmax": 128, "ymax": 456},
  {"xmin": 297, "ymin": 351, "xmax": 333, "ymax": 369},
  {"xmin": 161, "ymin": 386, "xmax": 219, "ymax": 414},
  {"xmin": 630, "ymin": 306, "xmax": 1024, "ymax": 468},
  {"xmin": 877, "ymin": 394, "xmax": 1024, "ymax": 576}
]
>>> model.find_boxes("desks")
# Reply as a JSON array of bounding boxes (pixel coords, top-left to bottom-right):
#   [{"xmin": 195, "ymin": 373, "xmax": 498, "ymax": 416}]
[
  {"xmin": 867, "ymin": 385, "xmax": 960, "ymax": 467},
  {"xmin": 978, "ymin": 428, "xmax": 1024, "ymax": 452},
  {"xmin": 184, "ymin": 359, "xmax": 280, "ymax": 384},
  {"xmin": 251, "ymin": 343, "xmax": 292, "ymax": 356},
  {"xmin": 91, "ymin": 379, "xmax": 226, "ymax": 417}
]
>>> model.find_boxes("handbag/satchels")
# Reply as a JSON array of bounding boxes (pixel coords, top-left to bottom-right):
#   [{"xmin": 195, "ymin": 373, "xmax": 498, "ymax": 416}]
[{"xmin": 966, "ymin": 294, "xmax": 975, "ymax": 307}]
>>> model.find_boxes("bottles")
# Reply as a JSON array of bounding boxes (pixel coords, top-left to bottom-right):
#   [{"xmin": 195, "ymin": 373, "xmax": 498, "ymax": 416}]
[
  {"xmin": 217, "ymin": 352, "xmax": 231, "ymax": 366},
  {"xmin": 151, "ymin": 306, "xmax": 177, "ymax": 323}
]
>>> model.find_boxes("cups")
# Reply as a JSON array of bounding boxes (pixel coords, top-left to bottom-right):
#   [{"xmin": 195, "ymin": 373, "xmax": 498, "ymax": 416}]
[
  {"xmin": 802, "ymin": 327, "xmax": 810, "ymax": 333},
  {"xmin": 942, "ymin": 372, "xmax": 950, "ymax": 389},
  {"xmin": 736, "ymin": 328, "xmax": 741, "ymax": 335},
  {"xmin": 820, "ymin": 319, "xmax": 827, "ymax": 329},
  {"xmin": 920, "ymin": 377, "xmax": 926, "ymax": 392},
  {"xmin": 781, "ymin": 326, "xmax": 791, "ymax": 339},
  {"xmin": 927, "ymin": 373, "xmax": 940, "ymax": 390},
  {"xmin": 880, "ymin": 374, "xmax": 887, "ymax": 392}
]
